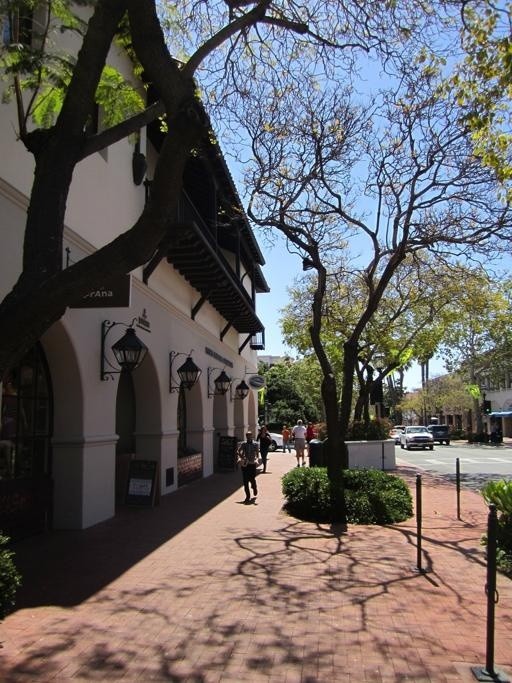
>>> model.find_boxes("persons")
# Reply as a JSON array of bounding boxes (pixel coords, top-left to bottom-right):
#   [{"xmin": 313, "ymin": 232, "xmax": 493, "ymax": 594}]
[
  {"xmin": 236, "ymin": 427, "xmax": 261, "ymax": 504},
  {"xmin": 305, "ymin": 420, "xmax": 316, "ymax": 444},
  {"xmin": 256, "ymin": 424, "xmax": 272, "ymax": 473},
  {"xmin": 291, "ymin": 418, "xmax": 308, "ymax": 468},
  {"xmin": 490, "ymin": 419, "xmax": 499, "ymax": 444},
  {"xmin": 281, "ymin": 423, "xmax": 292, "ymax": 454}
]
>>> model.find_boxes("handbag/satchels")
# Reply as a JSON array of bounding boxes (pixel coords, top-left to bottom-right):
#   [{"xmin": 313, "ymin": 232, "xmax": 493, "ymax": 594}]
[{"xmin": 235, "ymin": 455, "xmax": 249, "ymax": 467}]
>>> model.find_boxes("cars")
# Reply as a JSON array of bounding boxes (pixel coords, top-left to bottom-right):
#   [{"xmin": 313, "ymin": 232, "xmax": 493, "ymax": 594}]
[
  {"xmin": 390, "ymin": 425, "xmax": 405, "ymax": 442},
  {"xmin": 400, "ymin": 425, "xmax": 434, "ymax": 450},
  {"xmin": 257, "ymin": 426, "xmax": 286, "ymax": 452}
]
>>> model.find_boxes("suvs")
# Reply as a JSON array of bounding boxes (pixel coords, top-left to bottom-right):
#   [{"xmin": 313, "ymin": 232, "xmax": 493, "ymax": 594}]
[{"xmin": 427, "ymin": 424, "xmax": 451, "ymax": 446}]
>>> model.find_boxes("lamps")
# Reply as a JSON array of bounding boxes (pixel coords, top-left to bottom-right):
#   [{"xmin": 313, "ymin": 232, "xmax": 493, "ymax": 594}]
[
  {"xmin": 167, "ymin": 345, "xmax": 202, "ymax": 392},
  {"xmin": 230, "ymin": 375, "xmax": 250, "ymax": 399},
  {"xmin": 207, "ymin": 364, "xmax": 232, "ymax": 399},
  {"xmin": 99, "ymin": 308, "xmax": 153, "ymax": 381}
]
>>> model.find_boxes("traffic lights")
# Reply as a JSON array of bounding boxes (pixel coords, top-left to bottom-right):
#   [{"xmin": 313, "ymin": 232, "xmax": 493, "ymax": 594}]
[{"xmin": 484, "ymin": 400, "xmax": 492, "ymax": 414}]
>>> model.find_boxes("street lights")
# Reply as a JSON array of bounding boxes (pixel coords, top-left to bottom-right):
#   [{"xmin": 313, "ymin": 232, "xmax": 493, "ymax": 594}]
[{"xmin": 372, "ymin": 355, "xmax": 388, "ymax": 417}]
